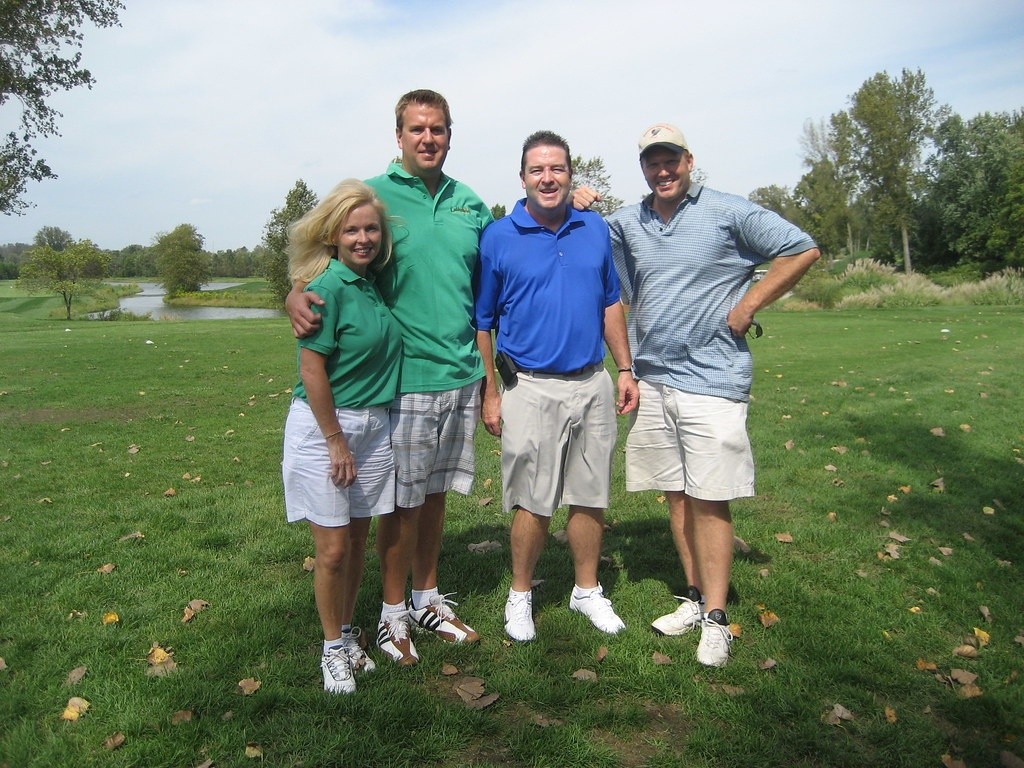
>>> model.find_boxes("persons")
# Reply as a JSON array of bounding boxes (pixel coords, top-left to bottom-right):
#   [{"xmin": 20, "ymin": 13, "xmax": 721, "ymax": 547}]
[
  {"xmin": 565, "ymin": 122, "xmax": 821, "ymax": 667},
  {"xmin": 472, "ymin": 131, "xmax": 640, "ymax": 643},
  {"xmin": 284, "ymin": 89, "xmax": 493, "ymax": 697}
]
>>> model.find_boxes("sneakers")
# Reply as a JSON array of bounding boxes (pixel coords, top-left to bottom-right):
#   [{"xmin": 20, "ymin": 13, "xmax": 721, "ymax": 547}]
[
  {"xmin": 696, "ymin": 609, "xmax": 733, "ymax": 667},
  {"xmin": 319, "ymin": 640, "xmax": 357, "ymax": 693},
  {"xmin": 503, "ymin": 590, "xmax": 537, "ymax": 640},
  {"xmin": 407, "ymin": 591, "xmax": 480, "ymax": 646},
  {"xmin": 342, "ymin": 626, "xmax": 375, "ymax": 674},
  {"xmin": 375, "ymin": 610, "xmax": 420, "ymax": 666},
  {"xmin": 569, "ymin": 581, "xmax": 625, "ymax": 634},
  {"xmin": 651, "ymin": 585, "xmax": 705, "ymax": 635}
]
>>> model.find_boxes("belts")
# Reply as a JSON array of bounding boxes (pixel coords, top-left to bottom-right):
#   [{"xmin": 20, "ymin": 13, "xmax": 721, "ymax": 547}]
[{"xmin": 512, "ymin": 362, "xmax": 601, "ymax": 376}]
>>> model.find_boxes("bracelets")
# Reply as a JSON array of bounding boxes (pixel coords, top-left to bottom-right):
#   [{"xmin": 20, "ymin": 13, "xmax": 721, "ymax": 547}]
[
  {"xmin": 617, "ymin": 368, "xmax": 631, "ymax": 374},
  {"xmin": 323, "ymin": 429, "xmax": 342, "ymax": 440}
]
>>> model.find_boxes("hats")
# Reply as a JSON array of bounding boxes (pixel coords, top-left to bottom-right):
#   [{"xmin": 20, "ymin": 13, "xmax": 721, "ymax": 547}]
[{"xmin": 638, "ymin": 123, "xmax": 689, "ymax": 160}]
